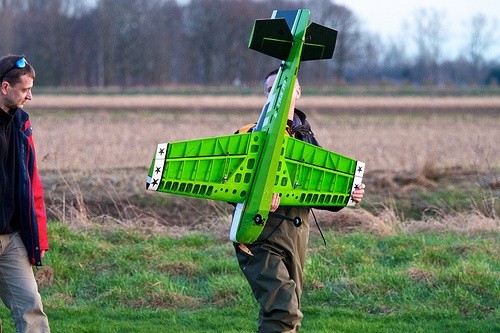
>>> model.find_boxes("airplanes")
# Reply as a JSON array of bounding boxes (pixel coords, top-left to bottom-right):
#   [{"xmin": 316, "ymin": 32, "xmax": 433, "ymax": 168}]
[{"xmin": 145, "ymin": 8, "xmax": 366, "ymax": 244}]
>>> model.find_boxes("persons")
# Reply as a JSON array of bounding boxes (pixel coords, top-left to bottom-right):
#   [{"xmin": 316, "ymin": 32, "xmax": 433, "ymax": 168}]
[
  {"xmin": 227, "ymin": 68, "xmax": 366, "ymax": 333},
  {"xmin": 0, "ymin": 55, "xmax": 52, "ymax": 333}
]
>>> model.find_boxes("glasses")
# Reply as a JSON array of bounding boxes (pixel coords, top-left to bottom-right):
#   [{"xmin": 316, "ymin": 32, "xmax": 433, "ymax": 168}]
[{"xmin": 0, "ymin": 58, "xmax": 28, "ymax": 84}]
